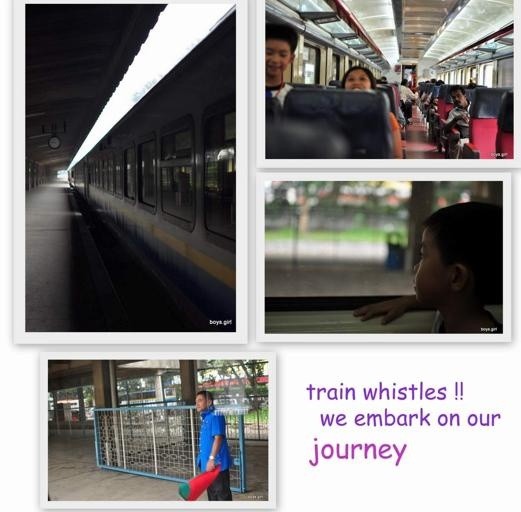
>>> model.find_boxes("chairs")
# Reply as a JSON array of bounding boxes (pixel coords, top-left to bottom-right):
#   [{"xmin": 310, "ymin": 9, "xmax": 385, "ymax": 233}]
[
  {"xmin": 417, "ymin": 80, "xmax": 514, "ymax": 159},
  {"xmin": 266, "ymin": 80, "xmax": 413, "ymax": 158}
]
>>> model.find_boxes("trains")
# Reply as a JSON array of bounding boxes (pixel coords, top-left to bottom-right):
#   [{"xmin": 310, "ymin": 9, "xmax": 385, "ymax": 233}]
[{"xmin": 67, "ymin": 4, "xmax": 236, "ymax": 332}]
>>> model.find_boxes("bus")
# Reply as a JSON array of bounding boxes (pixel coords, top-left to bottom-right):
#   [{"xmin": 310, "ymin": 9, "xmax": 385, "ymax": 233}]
[{"xmin": 119, "ymin": 387, "xmax": 177, "ymax": 417}]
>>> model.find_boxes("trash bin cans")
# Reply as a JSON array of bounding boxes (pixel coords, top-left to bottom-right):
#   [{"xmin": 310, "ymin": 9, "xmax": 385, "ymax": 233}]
[{"xmin": 388, "ymin": 243, "xmax": 405, "ymax": 270}]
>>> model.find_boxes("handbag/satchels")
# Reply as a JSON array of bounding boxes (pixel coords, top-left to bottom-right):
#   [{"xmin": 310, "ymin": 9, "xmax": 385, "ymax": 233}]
[{"xmin": 457, "ymin": 119, "xmax": 470, "ymax": 138}]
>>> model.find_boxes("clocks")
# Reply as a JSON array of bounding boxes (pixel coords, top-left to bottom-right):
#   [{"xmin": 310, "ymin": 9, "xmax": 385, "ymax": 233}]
[{"xmin": 48, "ymin": 131, "xmax": 62, "ymax": 151}]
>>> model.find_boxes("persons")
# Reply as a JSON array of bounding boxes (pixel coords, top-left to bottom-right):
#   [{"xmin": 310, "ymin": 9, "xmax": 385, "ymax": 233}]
[
  {"xmin": 353, "ymin": 199, "xmax": 502, "ymax": 332},
  {"xmin": 344, "ymin": 65, "xmax": 404, "ymax": 159},
  {"xmin": 195, "ymin": 391, "xmax": 235, "ymax": 500},
  {"xmin": 266, "ymin": 22, "xmax": 300, "ymax": 110},
  {"xmin": 423, "ymin": 79, "xmax": 443, "ymax": 113},
  {"xmin": 399, "ymin": 78, "xmax": 419, "ymax": 124},
  {"xmin": 468, "ymin": 79, "xmax": 477, "ymax": 89},
  {"xmin": 381, "ymin": 76, "xmax": 387, "ymax": 81},
  {"xmin": 437, "ymin": 85, "xmax": 474, "ymax": 159}
]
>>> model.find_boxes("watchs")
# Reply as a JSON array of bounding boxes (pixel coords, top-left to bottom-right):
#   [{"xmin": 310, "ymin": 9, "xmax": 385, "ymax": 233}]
[{"xmin": 209, "ymin": 455, "xmax": 216, "ymax": 459}]
